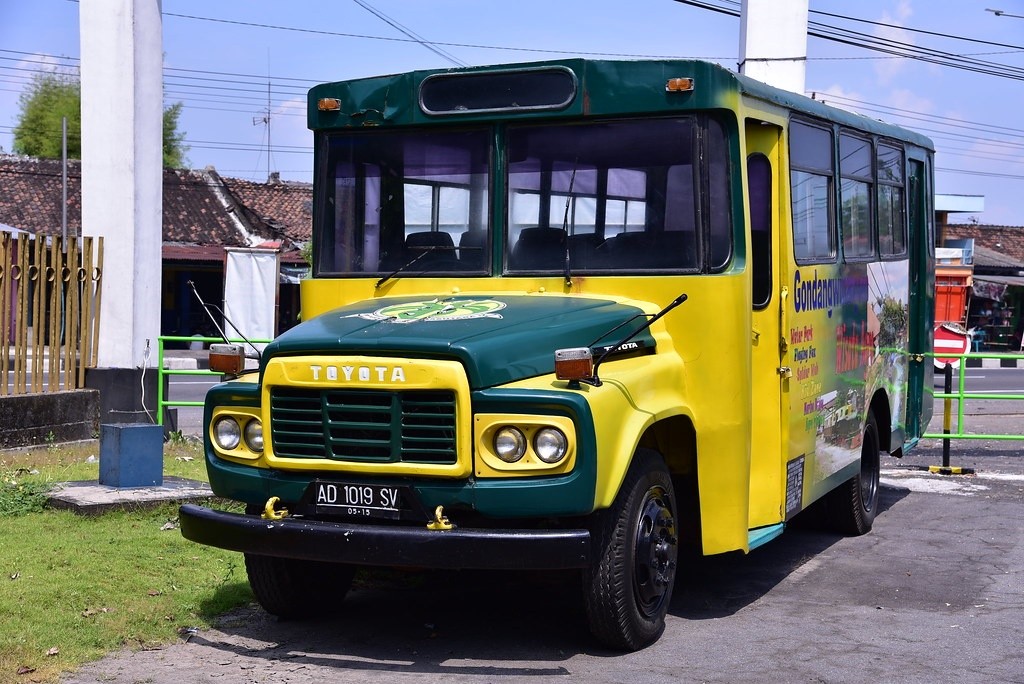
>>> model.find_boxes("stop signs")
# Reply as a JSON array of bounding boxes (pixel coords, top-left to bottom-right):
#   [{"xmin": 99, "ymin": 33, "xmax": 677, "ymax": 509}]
[{"xmin": 933, "ymin": 322, "xmax": 972, "ymax": 370}]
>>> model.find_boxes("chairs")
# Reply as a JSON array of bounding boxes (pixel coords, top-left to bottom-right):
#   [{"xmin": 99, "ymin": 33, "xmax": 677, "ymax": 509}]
[{"xmin": 400, "ymin": 227, "xmax": 698, "ymax": 266}]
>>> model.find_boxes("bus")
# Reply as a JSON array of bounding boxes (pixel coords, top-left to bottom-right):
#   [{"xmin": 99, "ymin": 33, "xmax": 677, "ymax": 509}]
[{"xmin": 179, "ymin": 58, "xmax": 935, "ymax": 650}]
